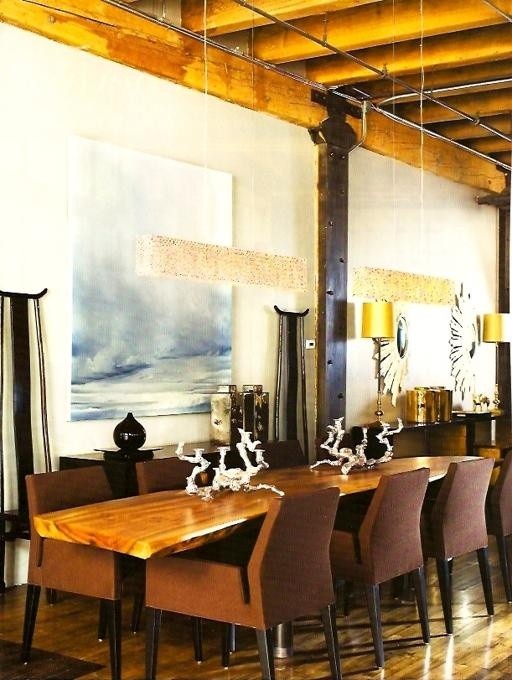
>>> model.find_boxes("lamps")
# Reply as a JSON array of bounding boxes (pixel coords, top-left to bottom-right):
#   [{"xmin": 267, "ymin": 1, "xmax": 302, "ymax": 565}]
[
  {"xmin": 360, "ymin": 297, "xmax": 396, "ymax": 427},
  {"xmin": 481, "ymin": 311, "xmax": 510, "ymax": 412}
]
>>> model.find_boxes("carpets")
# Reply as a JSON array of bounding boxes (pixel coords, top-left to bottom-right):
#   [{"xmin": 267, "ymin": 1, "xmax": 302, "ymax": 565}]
[{"xmin": 0, "ymin": 639, "xmax": 106, "ymax": 680}]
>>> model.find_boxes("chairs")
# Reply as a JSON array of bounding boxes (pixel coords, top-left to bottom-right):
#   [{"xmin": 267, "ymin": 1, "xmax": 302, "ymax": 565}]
[
  {"xmin": 34, "ymin": 455, "xmax": 489, "ymax": 658},
  {"xmin": 321, "ymin": 467, "xmax": 431, "ymax": 667},
  {"xmin": 392, "ymin": 457, "xmax": 495, "ymax": 634},
  {"xmin": 22, "ymin": 439, "xmax": 309, "ymax": 679},
  {"xmin": 146, "ymin": 487, "xmax": 342, "ymax": 678},
  {"xmin": 485, "ymin": 453, "xmax": 512, "ymax": 603}
]
